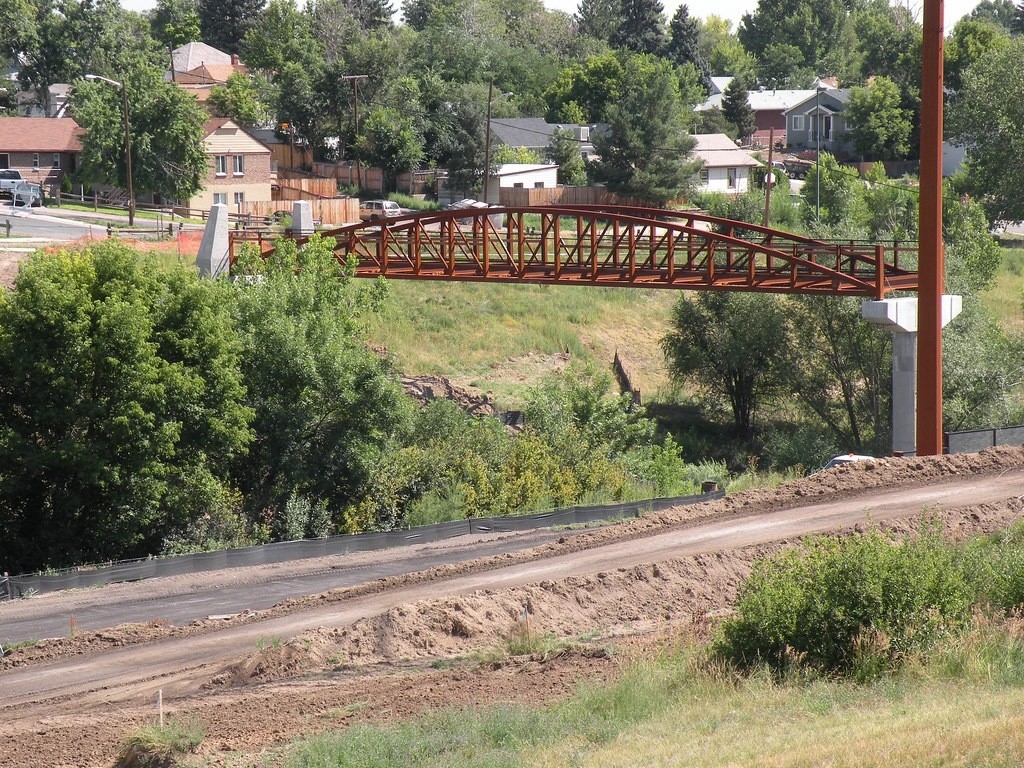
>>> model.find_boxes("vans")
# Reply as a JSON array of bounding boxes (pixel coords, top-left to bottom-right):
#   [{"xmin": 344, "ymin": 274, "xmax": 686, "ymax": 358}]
[
  {"xmin": 0, "ymin": 168, "xmax": 27, "ymax": 198},
  {"xmin": 359, "ymin": 199, "xmax": 402, "ymax": 226}
]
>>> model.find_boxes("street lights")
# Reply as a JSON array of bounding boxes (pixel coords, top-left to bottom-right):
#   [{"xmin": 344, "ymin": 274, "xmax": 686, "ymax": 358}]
[
  {"xmin": 86, "ymin": 74, "xmax": 135, "ymax": 225},
  {"xmin": 484, "ymin": 92, "xmax": 514, "ymax": 201}
]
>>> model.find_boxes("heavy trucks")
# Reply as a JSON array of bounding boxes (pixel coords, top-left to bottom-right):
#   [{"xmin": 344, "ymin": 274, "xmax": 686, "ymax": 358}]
[{"xmin": 764, "ymin": 158, "xmax": 815, "ymax": 180}]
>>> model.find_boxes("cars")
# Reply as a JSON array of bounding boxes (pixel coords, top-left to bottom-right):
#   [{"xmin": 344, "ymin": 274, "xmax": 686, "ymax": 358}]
[
  {"xmin": 10, "ymin": 182, "xmax": 46, "ymax": 207},
  {"xmin": 262, "ymin": 209, "xmax": 322, "ymax": 229}
]
